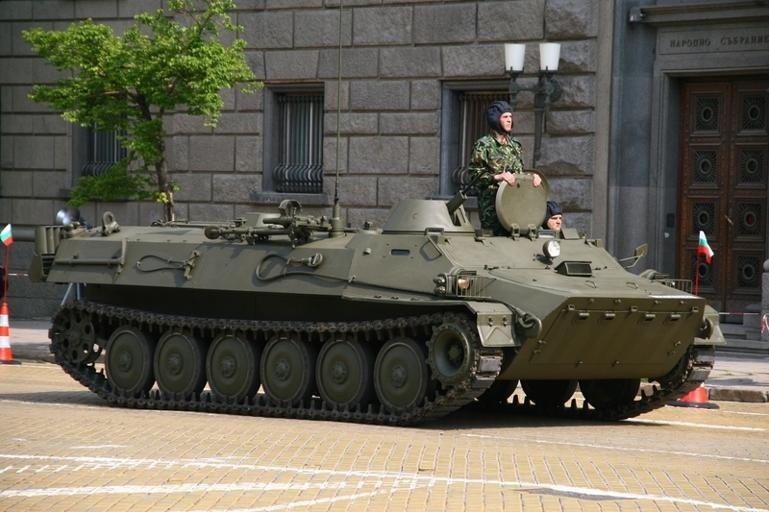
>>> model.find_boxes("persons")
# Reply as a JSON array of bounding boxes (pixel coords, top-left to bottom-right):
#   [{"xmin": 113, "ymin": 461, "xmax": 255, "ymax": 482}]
[
  {"xmin": 542, "ymin": 198, "xmax": 561, "ymax": 233},
  {"xmin": 463, "ymin": 101, "xmax": 543, "ymax": 236}
]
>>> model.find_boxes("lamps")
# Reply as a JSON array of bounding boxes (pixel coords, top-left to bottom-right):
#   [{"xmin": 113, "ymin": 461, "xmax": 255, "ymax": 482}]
[{"xmin": 504, "ymin": 43, "xmax": 560, "ymax": 163}]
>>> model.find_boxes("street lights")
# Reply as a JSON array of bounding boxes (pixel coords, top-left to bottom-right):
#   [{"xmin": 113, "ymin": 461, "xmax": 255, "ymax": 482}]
[{"xmin": 502, "ymin": 34, "xmax": 566, "ymax": 181}]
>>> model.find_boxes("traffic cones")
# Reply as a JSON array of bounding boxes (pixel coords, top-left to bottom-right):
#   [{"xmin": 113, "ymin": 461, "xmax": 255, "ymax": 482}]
[
  {"xmin": 668, "ymin": 379, "xmax": 720, "ymax": 411},
  {"xmin": 0, "ymin": 301, "xmax": 22, "ymax": 367}
]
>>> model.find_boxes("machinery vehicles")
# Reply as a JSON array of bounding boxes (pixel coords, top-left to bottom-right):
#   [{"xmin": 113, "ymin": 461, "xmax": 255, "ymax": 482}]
[{"xmin": 27, "ymin": 163, "xmax": 730, "ymax": 432}]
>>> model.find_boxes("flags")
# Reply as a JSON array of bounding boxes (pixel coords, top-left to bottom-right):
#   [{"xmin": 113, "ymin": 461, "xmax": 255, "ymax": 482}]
[
  {"xmin": 1, "ymin": 222, "xmax": 14, "ymax": 245},
  {"xmin": 697, "ymin": 231, "xmax": 713, "ymax": 264}
]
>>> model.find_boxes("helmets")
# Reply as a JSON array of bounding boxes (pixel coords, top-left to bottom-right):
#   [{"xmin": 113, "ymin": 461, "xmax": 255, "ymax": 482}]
[
  {"xmin": 542, "ymin": 201, "xmax": 562, "ymax": 229},
  {"xmin": 486, "ymin": 101, "xmax": 513, "ymax": 130}
]
